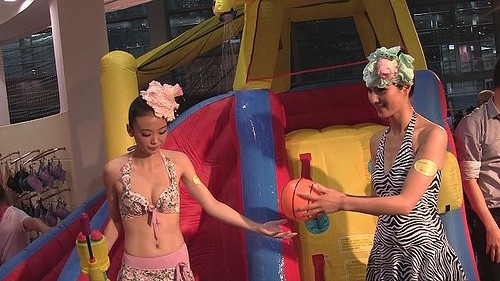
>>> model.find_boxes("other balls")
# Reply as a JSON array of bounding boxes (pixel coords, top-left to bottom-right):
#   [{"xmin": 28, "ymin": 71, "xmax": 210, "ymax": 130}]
[{"xmin": 280, "ymin": 178, "xmax": 322, "ymax": 223}]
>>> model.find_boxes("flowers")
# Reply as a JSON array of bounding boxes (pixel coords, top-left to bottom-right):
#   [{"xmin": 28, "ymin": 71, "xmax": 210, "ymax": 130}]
[
  {"xmin": 363, "ymin": 46, "xmax": 415, "ymax": 88},
  {"xmin": 139, "ymin": 79, "xmax": 184, "ymax": 122}
]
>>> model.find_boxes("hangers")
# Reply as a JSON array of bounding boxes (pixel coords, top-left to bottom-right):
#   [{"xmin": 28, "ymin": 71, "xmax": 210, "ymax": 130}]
[{"xmin": 8, "ymin": 150, "xmax": 66, "ymax": 213}]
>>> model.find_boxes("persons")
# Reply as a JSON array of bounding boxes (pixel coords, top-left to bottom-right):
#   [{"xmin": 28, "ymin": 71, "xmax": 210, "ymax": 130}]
[
  {"xmin": 454, "ymin": 59, "xmax": 500, "ymax": 281},
  {"xmin": 294, "ymin": 45, "xmax": 468, "ymax": 281},
  {"xmin": 103, "ymin": 80, "xmax": 298, "ymax": 281},
  {"xmin": 0, "ymin": 183, "xmax": 49, "ymax": 265}
]
